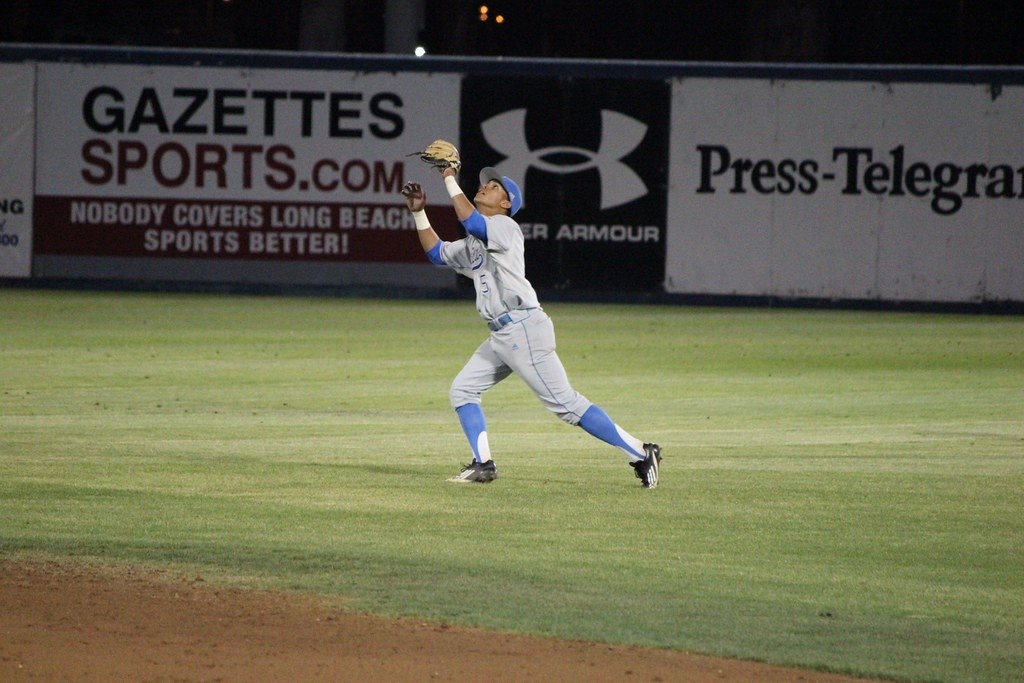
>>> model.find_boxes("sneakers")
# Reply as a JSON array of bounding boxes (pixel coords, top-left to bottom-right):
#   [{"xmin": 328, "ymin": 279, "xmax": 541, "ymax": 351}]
[
  {"xmin": 629, "ymin": 443, "xmax": 662, "ymax": 489},
  {"xmin": 445, "ymin": 458, "xmax": 497, "ymax": 483}
]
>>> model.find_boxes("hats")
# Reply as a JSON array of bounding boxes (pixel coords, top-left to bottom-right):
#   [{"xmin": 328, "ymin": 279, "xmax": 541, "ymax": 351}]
[{"xmin": 479, "ymin": 167, "xmax": 522, "ymax": 217}]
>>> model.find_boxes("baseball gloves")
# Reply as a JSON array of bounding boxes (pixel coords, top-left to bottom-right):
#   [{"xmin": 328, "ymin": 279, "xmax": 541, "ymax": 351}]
[{"xmin": 421, "ymin": 138, "xmax": 463, "ymax": 175}]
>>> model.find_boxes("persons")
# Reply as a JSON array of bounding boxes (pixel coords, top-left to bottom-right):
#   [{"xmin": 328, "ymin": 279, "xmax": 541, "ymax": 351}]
[{"xmin": 402, "ymin": 139, "xmax": 660, "ymax": 491}]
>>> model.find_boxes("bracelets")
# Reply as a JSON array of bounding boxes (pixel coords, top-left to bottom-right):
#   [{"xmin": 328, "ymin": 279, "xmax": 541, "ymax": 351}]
[
  {"xmin": 411, "ymin": 210, "xmax": 430, "ymax": 231},
  {"xmin": 444, "ymin": 176, "xmax": 463, "ymax": 198}
]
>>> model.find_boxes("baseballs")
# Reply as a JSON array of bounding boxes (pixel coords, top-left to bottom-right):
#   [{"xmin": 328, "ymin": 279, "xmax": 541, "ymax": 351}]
[{"xmin": 414, "ymin": 46, "xmax": 426, "ymax": 59}]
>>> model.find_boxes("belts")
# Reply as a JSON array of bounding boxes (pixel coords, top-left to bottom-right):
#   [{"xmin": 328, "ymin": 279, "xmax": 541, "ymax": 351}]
[{"xmin": 488, "ymin": 314, "xmax": 512, "ymax": 331}]
